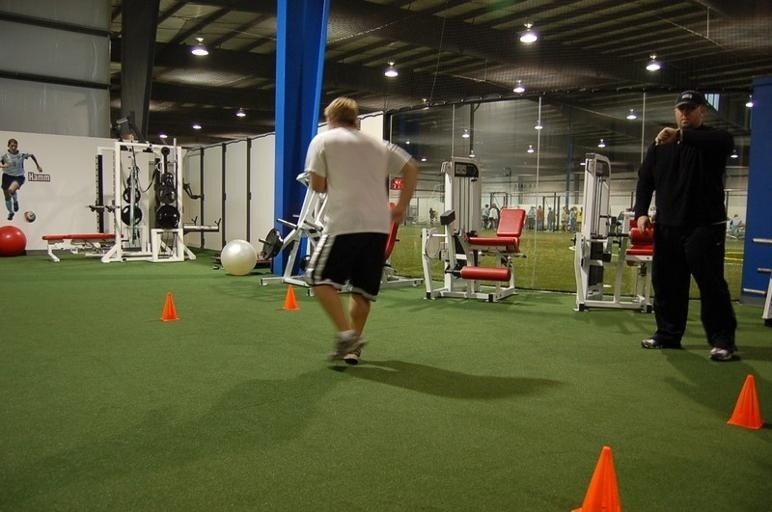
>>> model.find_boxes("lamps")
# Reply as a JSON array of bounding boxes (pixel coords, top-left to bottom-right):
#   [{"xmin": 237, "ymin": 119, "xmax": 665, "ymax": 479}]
[
  {"xmin": 597, "ymin": 138, "xmax": 606, "ymax": 148},
  {"xmin": 469, "ymin": 149, "xmax": 476, "ymax": 157},
  {"xmin": 462, "ymin": 128, "xmax": 470, "ymax": 138},
  {"xmin": 192, "ymin": 36, "xmax": 210, "ymax": 58},
  {"xmin": 645, "ymin": 53, "xmax": 663, "ymax": 72},
  {"xmin": 384, "ymin": 58, "xmax": 399, "ymax": 78},
  {"xmin": 534, "ymin": 120, "xmax": 544, "ymax": 130},
  {"xmin": 527, "ymin": 144, "xmax": 535, "ymax": 153},
  {"xmin": 626, "ymin": 109, "xmax": 638, "ymax": 121},
  {"xmin": 235, "ymin": 107, "xmax": 246, "ymax": 119},
  {"xmin": 519, "ymin": 18, "xmax": 538, "ymax": 44}
]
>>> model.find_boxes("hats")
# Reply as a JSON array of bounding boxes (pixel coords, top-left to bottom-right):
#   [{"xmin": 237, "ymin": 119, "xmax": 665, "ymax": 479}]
[{"xmin": 674, "ymin": 91, "xmax": 704, "ymax": 108}]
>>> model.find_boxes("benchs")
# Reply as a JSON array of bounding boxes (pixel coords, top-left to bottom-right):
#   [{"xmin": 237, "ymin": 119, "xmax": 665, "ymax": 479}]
[{"xmin": 41, "ymin": 232, "xmax": 124, "ymax": 263}]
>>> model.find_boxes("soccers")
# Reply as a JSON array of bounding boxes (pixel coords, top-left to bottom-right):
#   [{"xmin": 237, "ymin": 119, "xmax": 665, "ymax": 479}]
[{"xmin": 24, "ymin": 209, "xmax": 36, "ymax": 222}]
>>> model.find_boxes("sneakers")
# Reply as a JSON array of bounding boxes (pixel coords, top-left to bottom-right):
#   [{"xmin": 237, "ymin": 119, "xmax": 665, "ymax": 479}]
[
  {"xmin": 8, "ymin": 201, "xmax": 18, "ymax": 220},
  {"xmin": 327, "ymin": 333, "xmax": 362, "ymax": 363},
  {"xmin": 641, "ymin": 337, "xmax": 667, "ymax": 349},
  {"xmin": 710, "ymin": 347, "xmax": 732, "ymax": 360}
]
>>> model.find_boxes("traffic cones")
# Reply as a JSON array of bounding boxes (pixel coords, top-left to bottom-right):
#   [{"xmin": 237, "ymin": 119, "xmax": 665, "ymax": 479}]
[
  {"xmin": 159, "ymin": 292, "xmax": 180, "ymax": 322},
  {"xmin": 572, "ymin": 446, "xmax": 624, "ymax": 512},
  {"xmin": 282, "ymin": 284, "xmax": 302, "ymax": 311},
  {"xmin": 727, "ymin": 374, "xmax": 768, "ymax": 430}
]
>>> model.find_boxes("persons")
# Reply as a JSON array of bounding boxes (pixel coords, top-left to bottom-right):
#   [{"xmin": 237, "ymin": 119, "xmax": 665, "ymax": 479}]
[
  {"xmin": 304, "ymin": 97, "xmax": 420, "ymax": 366},
  {"xmin": 729, "ymin": 212, "xmax": 741, "ymax": 240},
  {"xmin": 482, "ymin": 200, "xmax": 584, "ymax": 232},
  {"xmin": 0, "ymin": 137, "xmax": 43, "ymax": 220},
  {"xmin": 630, "ymin": 86, "xmax": 743, "ymax": 362}
]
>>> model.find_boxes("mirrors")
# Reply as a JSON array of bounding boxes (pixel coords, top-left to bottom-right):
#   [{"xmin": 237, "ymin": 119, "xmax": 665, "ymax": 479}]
[{"xmin": 386, "ymin": 87, "xmax": 754, "ymax": 303}]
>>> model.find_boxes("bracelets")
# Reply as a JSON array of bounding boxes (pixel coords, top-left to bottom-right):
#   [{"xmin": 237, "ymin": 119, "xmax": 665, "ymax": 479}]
[{"xmin": 674, "ymin": 127, "xmax": 680, "ymax": 146}]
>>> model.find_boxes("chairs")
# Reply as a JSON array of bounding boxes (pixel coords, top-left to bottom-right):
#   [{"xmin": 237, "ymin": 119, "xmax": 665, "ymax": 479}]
[
  {"xmin": 457, "ymin": 208, "xmax": 526, "ymax": 302},
  {"xmin": 623, "ymin": 216, "xmax": 656, "ymax": 313}
]
